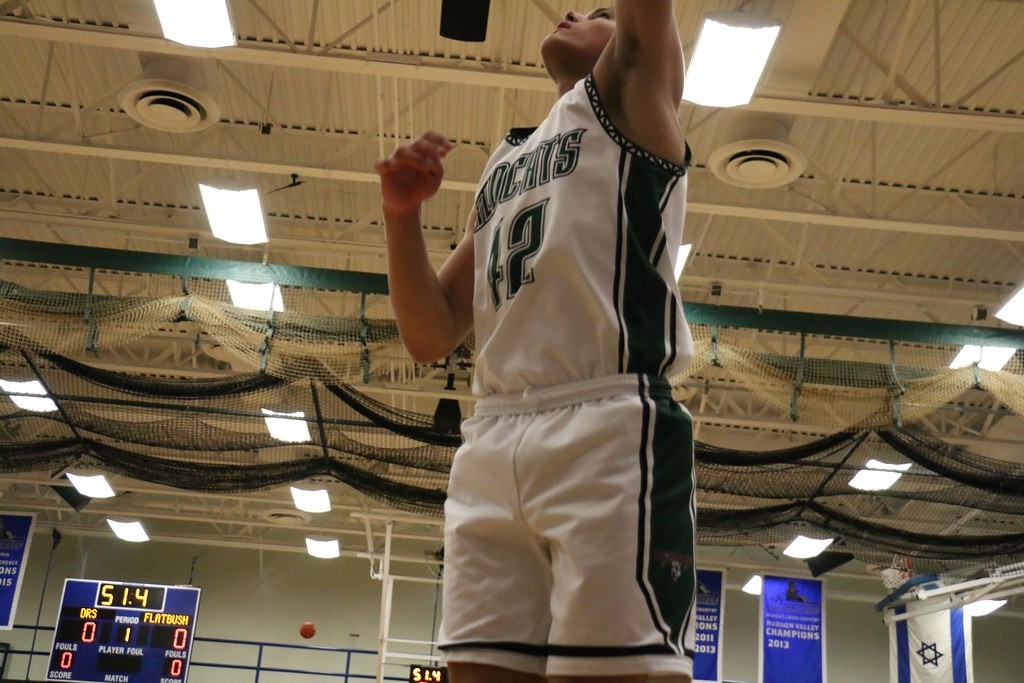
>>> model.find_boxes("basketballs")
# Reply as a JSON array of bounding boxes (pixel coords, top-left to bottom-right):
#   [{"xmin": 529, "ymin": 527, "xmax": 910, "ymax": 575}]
[{"xmin": 299, "ymin": 622, "xmax": 317, "ymax": 639}]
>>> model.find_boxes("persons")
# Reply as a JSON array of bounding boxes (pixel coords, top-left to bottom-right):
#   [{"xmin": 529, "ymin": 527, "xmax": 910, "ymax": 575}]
[{"xmin": 374, "ymin": 0, "xmax": 697, "ymax": 683}]
